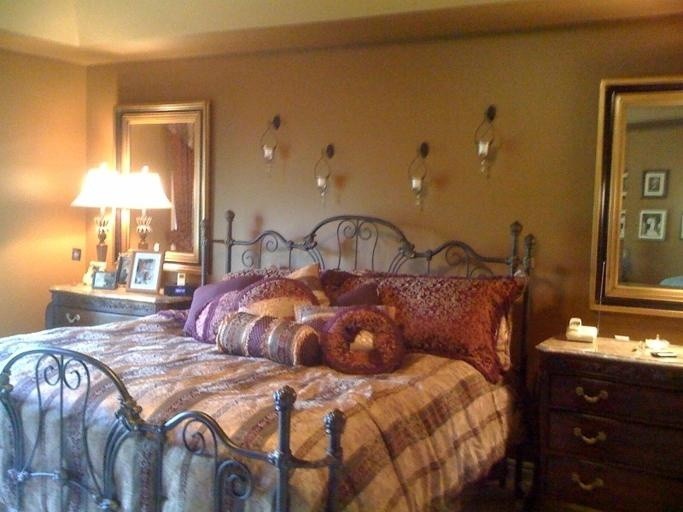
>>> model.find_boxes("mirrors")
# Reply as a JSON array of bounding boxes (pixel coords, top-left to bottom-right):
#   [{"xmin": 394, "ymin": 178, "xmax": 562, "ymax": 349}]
[
  {"xmin": 115, "ymin": 98, "xmax": 212, "ymax": 277},
  {"xmin": 589, "ymin": 76, "xmax": 683, "ymax": 319}
]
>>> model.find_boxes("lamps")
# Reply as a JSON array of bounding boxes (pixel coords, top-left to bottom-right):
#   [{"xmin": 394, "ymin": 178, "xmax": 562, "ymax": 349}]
[
  {"xmin": 408, "ymin": 141, "xmax": 429, "ymax": 212},
  {"xmin": 259, "ymin": 115, "xmax": 280, "ymax": 164},
  {"xmin": 474, "ymin": 105, "xmax": 496, "ymax": 177},
  {"xmin": 122, "ymin": 165, "xmax": 173, "ymax": 251},
  {"xmin": 314, "ymin": 145, "xmax": 334, "ymax": 202},
  {"xmin": 68, "ymin": 162, "xmax": 122, "ymax": 262}
]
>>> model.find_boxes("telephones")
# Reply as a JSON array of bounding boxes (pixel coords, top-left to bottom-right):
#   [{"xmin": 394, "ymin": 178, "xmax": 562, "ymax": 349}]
[{"xmin": 566, "ymin": 262, "xmax": 606, "ymax": 342}]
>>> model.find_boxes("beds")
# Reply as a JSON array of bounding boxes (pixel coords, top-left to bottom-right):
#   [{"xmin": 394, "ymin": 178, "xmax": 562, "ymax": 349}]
[{"xmin": 0, "ymin": 210, "xmax": 536, "ymax": 512}]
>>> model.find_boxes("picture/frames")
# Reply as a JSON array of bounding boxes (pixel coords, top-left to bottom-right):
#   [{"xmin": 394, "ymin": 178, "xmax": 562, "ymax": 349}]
[
  {"xmin": 638, "ymin": 208, "xmax": 668, "ymax": 242},
  {"xmin": 83, "ymin": 250, "xmax": 165, "ymax": 295},
  {"xmin": 640, "ymin": 169, "xmax": 669, "ymax": 200}
]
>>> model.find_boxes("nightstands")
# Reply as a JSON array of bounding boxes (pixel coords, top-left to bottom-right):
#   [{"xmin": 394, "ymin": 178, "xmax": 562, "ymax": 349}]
[
  {"xmin": 45, "ymin": 285, "xmax": 193, "ymax": 329},
  {"xmin": 533, "ymin": 332, "xmax": 683, "ymax": 511}
]
